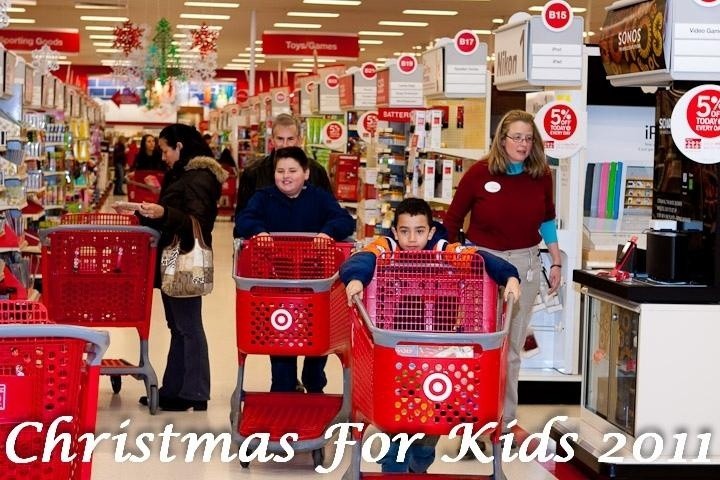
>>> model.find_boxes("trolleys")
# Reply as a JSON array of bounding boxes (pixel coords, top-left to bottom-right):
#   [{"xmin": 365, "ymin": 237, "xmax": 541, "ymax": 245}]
[
  {"xmin": 124, "ymin": 170, "xmax": 165, "ymax": 204},
  {"xmin": 343, "ymin": 285, "xmax": 508, "ymax": 480},
  {"xmin": 228, "ymin": 232, "xmax": 360, "ymax": 470},
  {"xmin": 215, "ymin": 163, "xmax": 237, "ymax": 221},
  {"xmin": 38, "ymin": 212, "xmax": 161, "ymax": 415},
  {"xmin": 1, "ymin": 323, "xmax": 109, "ymax": 480}
]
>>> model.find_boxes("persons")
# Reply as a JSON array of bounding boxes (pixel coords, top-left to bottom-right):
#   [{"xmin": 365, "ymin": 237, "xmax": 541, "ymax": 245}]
[
  {"xmin": 112, "ymin": 133, "xmax": 236, "ymax": 194},
  {"xmin": 110, "ymin": 123, "xmax": 230, "ymax": 410},
  {"xmin": 443, "ymin": 109, "xmax": 563, "ymax": 458},
  {"xmin": 237, "ymin": 112, "xmax": 333, "ymax": 204},
  {"xmin": 338, "ymin": 197, "xmax": 522, "ymax": 473},
  {"xmin": 235, "ymin": 147, "xmax": 355, "ymax": 393}
]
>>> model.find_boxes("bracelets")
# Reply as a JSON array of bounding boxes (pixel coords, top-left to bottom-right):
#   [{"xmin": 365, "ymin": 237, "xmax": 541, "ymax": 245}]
[{"xmin": 549, "ymin": 263, "xmax": 563, "ymax": 269}]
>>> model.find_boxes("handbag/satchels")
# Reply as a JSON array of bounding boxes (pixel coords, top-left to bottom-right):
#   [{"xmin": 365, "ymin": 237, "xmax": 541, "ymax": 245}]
[{"xmin": 160, "ymin": 215, "xmax": 213, "ymax": 298}]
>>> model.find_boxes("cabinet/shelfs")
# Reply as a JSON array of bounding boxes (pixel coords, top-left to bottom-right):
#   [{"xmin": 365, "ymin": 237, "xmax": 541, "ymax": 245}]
[{"xmin": 550, "ymin": 267, "xmax": 720, "ymax": 479}]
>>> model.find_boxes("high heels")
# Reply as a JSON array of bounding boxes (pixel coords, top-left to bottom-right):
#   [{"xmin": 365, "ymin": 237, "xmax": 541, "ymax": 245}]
[
  {"xmin": 139, "ymin": 387, "xmax": 179, "ymax": 406},
  {"xmin": 162, "ymin": 397, "xmax": 207, "ymax": 411}
]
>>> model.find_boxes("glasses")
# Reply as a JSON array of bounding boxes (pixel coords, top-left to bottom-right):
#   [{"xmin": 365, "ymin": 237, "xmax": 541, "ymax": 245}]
[{"xmin": 506, "ymin": 135, "xmax": 533, "ymax": 143}]
